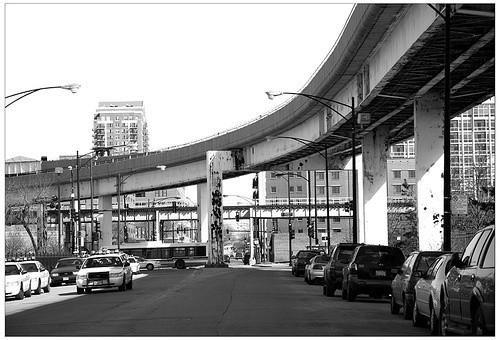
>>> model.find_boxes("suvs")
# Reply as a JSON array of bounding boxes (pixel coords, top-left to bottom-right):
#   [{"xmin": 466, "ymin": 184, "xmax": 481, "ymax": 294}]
[
  {"xmin": 291, "ymin": 250, "xmax": 320, "ymax": 277},
  {"xmin": 440, "ymin": 225, "xmax": 494, "ymax": 336},
  {"xmin": 342, "ymin": 244, "xmax": 405, "ymax": 301},
  {"xmin": 322, "ymin": 244, "xmax": 366, "ymax": 296}
]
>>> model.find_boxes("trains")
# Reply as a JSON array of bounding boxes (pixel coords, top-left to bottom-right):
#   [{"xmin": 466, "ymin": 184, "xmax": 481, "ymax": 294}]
[{"xmin": 60, "ymin": 199, "xmax": 188, "ymax": 210}]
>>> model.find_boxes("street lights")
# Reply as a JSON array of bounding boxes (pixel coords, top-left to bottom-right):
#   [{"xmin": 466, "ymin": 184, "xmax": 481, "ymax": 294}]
[
  {"xmin": 118, "ymin": 164, "xmax": 167, "ymax": 249},
  {"xmin": 265, "ymin": 91, "xmax": 356, "ymax": 244},
  {"xmin": 223, "ymin": 195, "xmax": 258, "ymax": 263},
  {"xmin": 67, "ymin": 165, "xmax": 75, "ymax": 253},
  {"xmin": 77, "ymin": 143, "xmax": 138, "ymax": 255},
  {"xmin": 266, "ymin": 134, "xmax": 330, "ymax": 251},
  {"xmin": 5, "ymin": 83, "xmax": 83, "ymax": 110},
  {"xmin": 276, "ymin": 169, "xmax": 312, "ymax": 249},
  {"xmin": 147, "ymin": 196, "xmax": 181, "ymax": 240}
]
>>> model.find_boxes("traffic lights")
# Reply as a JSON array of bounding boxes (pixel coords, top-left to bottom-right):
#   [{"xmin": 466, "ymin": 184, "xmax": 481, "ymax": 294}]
[
  {"xmin": 53, "ymin": 195, "xmax": 57, "ymax": 200},
  {"xmin": 124, "ymin": 226, "xmax": 127, "ymax": 239},
  {"xmin": 236, "ymin": 211, "xmax": 239, "ymax": 222},
  {"xmin": 75, "ymin": 212, "xmax": 79, "ymax": 222}
]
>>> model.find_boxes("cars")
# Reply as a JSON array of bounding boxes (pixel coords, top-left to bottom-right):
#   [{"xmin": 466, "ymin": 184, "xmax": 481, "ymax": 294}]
[
  {"xmin": 75, "ymin": 256, "xmax": 132, "ymax": 293},
  {"xmin": 130, "ymin": 255, "xmax": 158, "ymax": 271},
  {"xmin": 411, "ymin": 253, "xmax": 463, "ymax": 335},
  {"xmin": 390, "ymin": 251, "xmax": 453, "ymax": 319},
  {"xmin": 50, "ymin": 258, "xmax": 83, "ymax": 286},
  {"xmin": 303, "ymin": 254, "xmax": 330, "ymax": 285},
  {"xmin": 19, "ymin": 260, "xmax": 52, "ymax": 294},
  {"xmin": 5, "ymin": 262, "xmax": 31, "ymax": 300}
]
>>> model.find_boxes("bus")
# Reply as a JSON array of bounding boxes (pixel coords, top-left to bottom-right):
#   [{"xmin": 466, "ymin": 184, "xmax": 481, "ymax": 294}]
[{"xmin": 102, "ymin": 245, "xmax": 209, "ymax": 269}]
